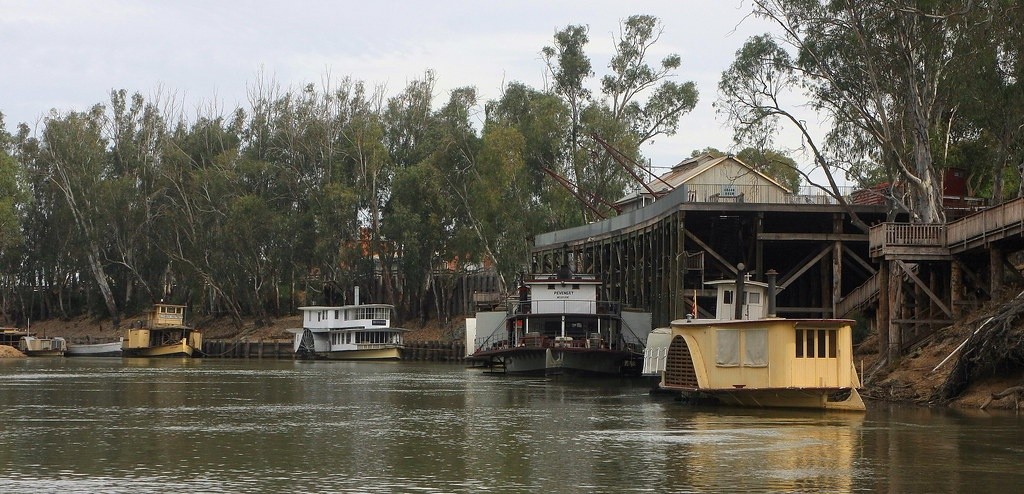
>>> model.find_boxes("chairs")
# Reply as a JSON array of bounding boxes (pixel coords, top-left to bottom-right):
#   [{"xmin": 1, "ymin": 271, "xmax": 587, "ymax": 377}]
[
  {"xmin": 525, "ymin": 332, "xmax": 541, "ymax": 347},
  {"xmin": 586, "ymin": 333, "xmax": 603, "ymax": 349}
]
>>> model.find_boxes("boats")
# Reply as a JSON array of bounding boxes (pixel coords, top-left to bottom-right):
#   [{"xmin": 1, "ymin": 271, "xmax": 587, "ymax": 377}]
[
  {"xmin": 292, "ymin": 285, "xmax": 412, "ymax": 361},
  {"xmin": 117, "ymin": 298, "xmax": 203, "ymax": 358},
  {"xmin": 21, "ymin": 318, "xmax": 70, "ymax": 358},
  {"xmin": 637, "ymin": 263, "xmax": 869, "ymax": 412},
  {"xmin": 465, "ymin": 265, "xmax": 639, "ymax": 376}
]
